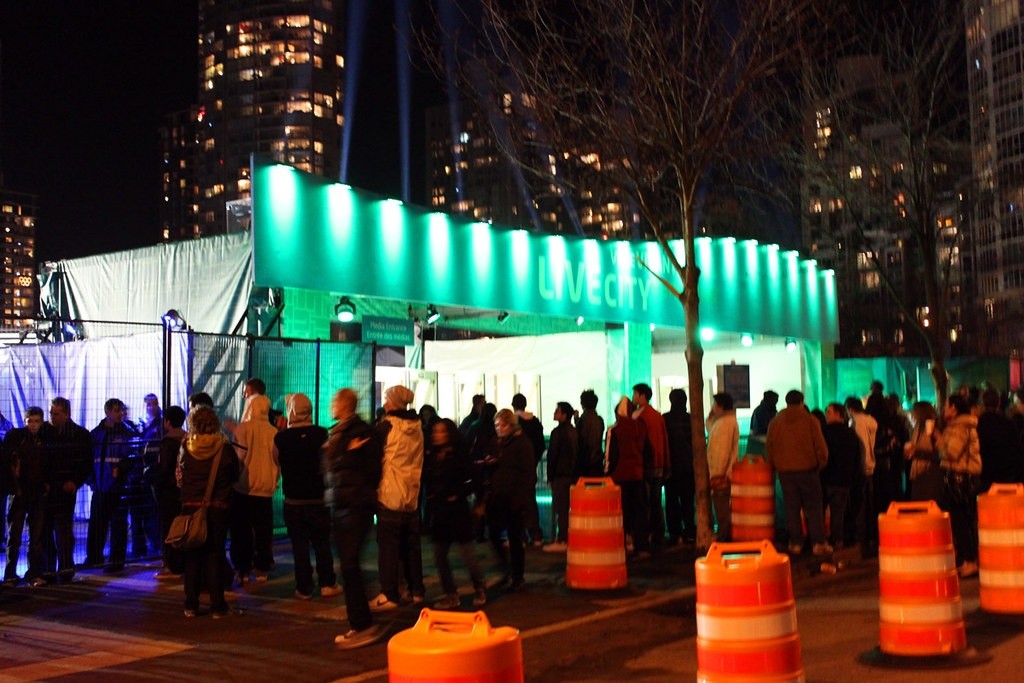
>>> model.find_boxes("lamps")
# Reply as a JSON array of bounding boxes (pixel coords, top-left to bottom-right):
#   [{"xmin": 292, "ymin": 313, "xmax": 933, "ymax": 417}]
[
  {"xmin": 334, "ymin": 296, "xmax": 356, "ymax": 322},
  {"xmin": 426, "ymin": 304, "xmax": 440, "ymax": 324},
  {"xmin": 497, "ymin": 311, "xmax": 510, "ymax": 324},
  {"xmin": 740, "ymin": 333, "xmax": 753, "ymax": 346},
  {"xmin": 785, "ymin": 337, "xmax": 796, "ymax": 353}
]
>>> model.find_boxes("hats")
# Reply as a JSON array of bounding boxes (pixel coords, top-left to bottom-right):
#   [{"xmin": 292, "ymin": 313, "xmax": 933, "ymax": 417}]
[
  {"xmin": 284, "ymin": 392, "xmax": 312, "ymax": 422},
  {"xmin": 388, "ymin": 386, "xmax": 415, "ymax": 411}
]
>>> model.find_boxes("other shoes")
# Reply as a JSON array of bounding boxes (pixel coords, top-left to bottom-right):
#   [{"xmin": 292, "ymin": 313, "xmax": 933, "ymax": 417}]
[
  {"xmin": 184, "ymin": 609, "xmax": 195, "ymax": 618},
  {"xmin": 334, "ymin": 626, "xmax": 384, "ymax": 648},
  {"xmin": 2, "ymin": 547, "xmax": 164, "ymax": 585},
  {"xmin": 149, "ymin": 567, "xmax": 525, "ymax": 609},
  {"xmin": 210, "ymin": 607, "xmax": 241, "ymax": 619},
  {"xmin": 623, "ymin": 533, "xmax": 696, "ymax": 560},
  {"xmin": 785, "ymin": 543, "xmax": 877, "ymax": 558},
  {"xmin": 956, "ymin": 567, "xmax": 979, "ymax": 578},
  {"xmin": 502, "ymin": 536, "xmax": 567, "ymax": 552}
]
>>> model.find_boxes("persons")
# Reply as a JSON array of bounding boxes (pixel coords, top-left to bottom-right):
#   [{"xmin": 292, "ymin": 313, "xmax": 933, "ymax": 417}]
[{"xmin": 0, "ymin": 374, "xmax": 1018, "ymax": 653}]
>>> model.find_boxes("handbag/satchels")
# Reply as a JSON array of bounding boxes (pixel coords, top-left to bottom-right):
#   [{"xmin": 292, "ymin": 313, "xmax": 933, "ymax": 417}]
[
  {"xmin": 942, "ymin": 473, "xmax": 970, "ymax": 516},
  {"xmin": 709, "ymin": 474, "xmax": 731, "ymax": 503},
  {"xmin": 164, "ymin": 507, "xmax": 207, "ymax": 551}
]
